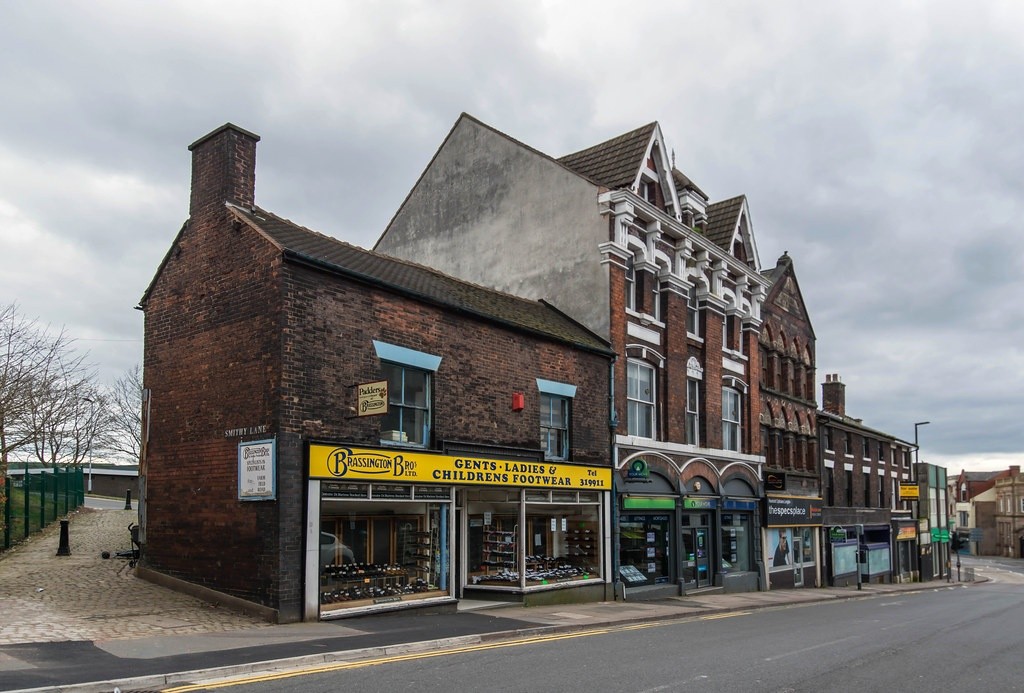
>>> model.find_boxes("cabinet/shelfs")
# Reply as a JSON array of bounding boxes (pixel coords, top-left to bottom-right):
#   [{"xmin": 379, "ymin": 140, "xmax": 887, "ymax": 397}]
[
  {"xmin": 563, "ymin": 531, "xmax": 598, "ymax": 557},
  {"xmin": 485, "ymin": 524, "xmax": 518, "ymax": 576},
  {"xmin": 402, "ymin": 523, "xmax": 435, "ymax": 584}
]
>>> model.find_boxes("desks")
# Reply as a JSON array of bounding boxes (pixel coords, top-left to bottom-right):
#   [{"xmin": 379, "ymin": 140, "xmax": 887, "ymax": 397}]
[
  {"xmin": 526, "ymin": 560, "xmax": 559, "ymax": 572},
  {"xmin": 343, "ymin": 576, "xmax": 405, "ymax": 594}
]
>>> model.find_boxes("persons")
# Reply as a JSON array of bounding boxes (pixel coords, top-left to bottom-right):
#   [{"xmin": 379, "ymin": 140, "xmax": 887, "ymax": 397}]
[{"xmin": 773, "ymin": 527, "xmax": 792, "ymax": 567}]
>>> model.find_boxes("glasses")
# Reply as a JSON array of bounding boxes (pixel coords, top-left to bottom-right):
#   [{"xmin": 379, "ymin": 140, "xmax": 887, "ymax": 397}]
[{"xmin": 781, "ymin": 536, "xmax": 787, "ymax": 540}]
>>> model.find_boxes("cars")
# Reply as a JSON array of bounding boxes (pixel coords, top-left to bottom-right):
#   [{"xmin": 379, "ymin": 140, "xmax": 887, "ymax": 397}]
[{"xmin": 320, "ymin": 531, "xmax": 353, "ymax": 575}]
